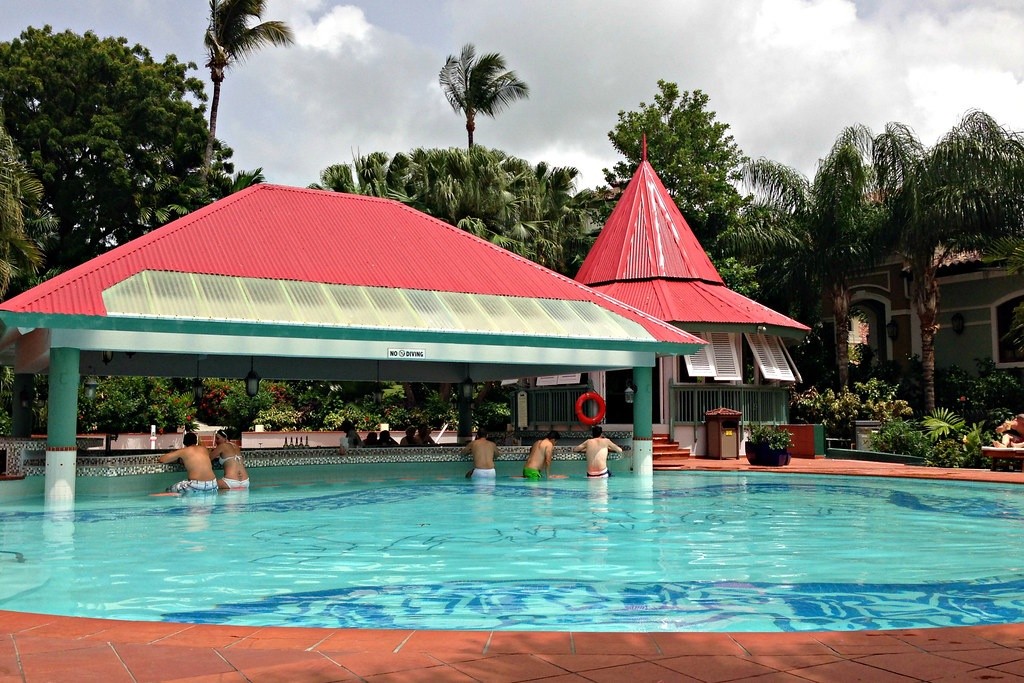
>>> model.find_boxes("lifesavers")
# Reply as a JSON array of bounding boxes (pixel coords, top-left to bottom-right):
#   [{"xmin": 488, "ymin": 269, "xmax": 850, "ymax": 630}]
[{"xmin": 575, "ymin": 391, "xmax": 606, "ymax": 426}]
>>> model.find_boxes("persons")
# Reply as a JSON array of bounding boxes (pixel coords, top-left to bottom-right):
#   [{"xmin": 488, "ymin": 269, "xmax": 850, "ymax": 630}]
[
  {"xmin": 400, "ymin": 427, "xmax": 419, "ymax": 447},
  {"xmin": 992, "ymin": 415, "xmax": 1024, "ymax": 448},
  {"xmin": 378, "ymin": 430, "xmax": 399, "ymax": 447},
  {"xmin": 160, "ymin": 432, "xmax": 219, "ymax": 493},
  {"xmin": 573, "ymin": 426, "xmax": 623, "ymax": 478},
  {"xmin": 361, "ymin": 432, "xmax": 380, "ymax": 448},
  {"xmin": 210, "ymin": 429, "xmax": 250, "ymax": 490},
  {"xmin": 521, "ymin": 430, "xmax": 560, "ymax": 480},
  {"xmin": 461, "ymin": 428, "xmax": 500, "ymax": 479},
  {"xmin": 345, "ymin": 430, "xmax": 364, "ymax": 448},
  {"xmin": 415, "ymin": 425, "xmax": 436, "ymax": 446}
]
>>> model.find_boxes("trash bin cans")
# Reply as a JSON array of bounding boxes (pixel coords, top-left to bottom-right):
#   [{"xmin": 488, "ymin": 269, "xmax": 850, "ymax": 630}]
[
  {"xmin": 854, "ymin": 420, "xmax": 881, "ymax": 452},
  {"xmin": 704, "ymin": 406, "xmax": 743, "ymax": 460}
]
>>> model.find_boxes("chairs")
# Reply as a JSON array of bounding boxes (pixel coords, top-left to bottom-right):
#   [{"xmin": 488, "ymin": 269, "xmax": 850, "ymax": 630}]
[{"xmin": 981, "ymin": 446, "xmax": 1024, "ymax": 472}]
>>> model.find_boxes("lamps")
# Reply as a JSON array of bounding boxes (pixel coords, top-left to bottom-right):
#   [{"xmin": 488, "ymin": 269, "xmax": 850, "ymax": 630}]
[
  {"xmin": 243, "ymin": 356, "xmax": 261, "ymax": 400},
  {"xmin": 624, "ymin": 385, "xmax": 634, "ymax": 403},
  {"xmin": 460, "ymin": 363, "xmax": 473, "ymax": 401}
]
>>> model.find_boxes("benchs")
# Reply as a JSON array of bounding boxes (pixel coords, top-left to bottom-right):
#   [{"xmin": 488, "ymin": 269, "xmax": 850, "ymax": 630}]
[{"xmin": 198, "ymin": 436, "xmax": 242, "ymax": 449}]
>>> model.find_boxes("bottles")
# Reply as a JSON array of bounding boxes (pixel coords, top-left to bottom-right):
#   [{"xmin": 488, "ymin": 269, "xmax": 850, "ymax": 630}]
[
  {"xmin": 289, "ymin": 437, "xmax": 295, "ymax": 449},
  {"xmin": 305, "ymin": 436, "xmax": 309, "ymax": 449},
  {"xmin": 294, "ymin": 437, "xmax": 298, "ymax": 448},
  {"xmin": 298, "ymin": 437, "xmax": 304, "ymax": 448},
  {"xmin": 283, "ymin": 437, "xmax": 289, "ymax": 449}
]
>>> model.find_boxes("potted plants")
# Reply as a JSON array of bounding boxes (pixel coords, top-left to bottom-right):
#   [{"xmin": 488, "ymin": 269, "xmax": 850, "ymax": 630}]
[{"xmin": 744, "ymin": 421, "xmax": 795, "ymax": 466}]
